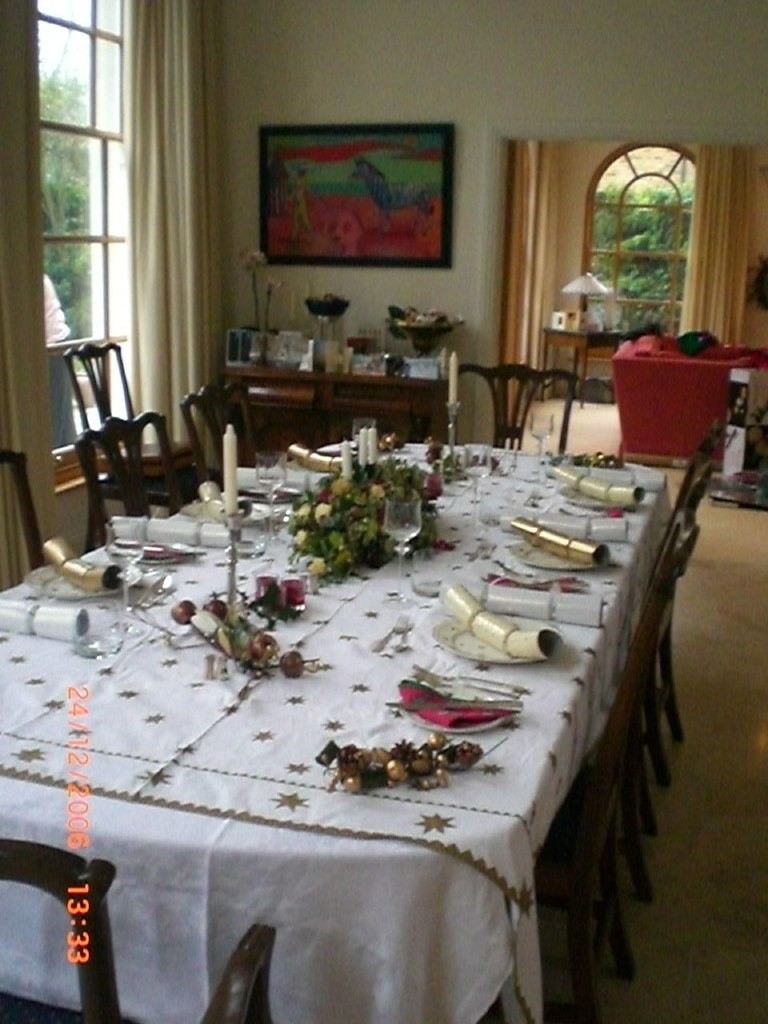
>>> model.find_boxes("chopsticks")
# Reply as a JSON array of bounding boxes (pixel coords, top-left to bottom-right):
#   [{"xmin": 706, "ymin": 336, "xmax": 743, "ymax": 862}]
[{"xmin": 386, "ymin": 702, "xmax": 524, "ymax": 709}]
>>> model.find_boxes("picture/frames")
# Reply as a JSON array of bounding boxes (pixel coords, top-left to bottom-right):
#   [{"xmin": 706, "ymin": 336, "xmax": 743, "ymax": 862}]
[{"xmin": 258, "ymin": 122, "xmax": 454, "ymax": 270}]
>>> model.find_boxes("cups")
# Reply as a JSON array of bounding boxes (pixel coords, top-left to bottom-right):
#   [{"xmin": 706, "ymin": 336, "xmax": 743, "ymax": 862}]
[
  {"xmin": 351, "ymin": 418, "xmax": 376, "ymax": 448},
  {"xmin": 413, "ymin": 547, "xmax": 444, "ymax": 597},
  {"xmin": 71, "ymin": 599, "xmax": 125, "ymax": 660},
  {"xmin": 279, "ymin": 577, "xmax": 305, "ymax": 611},
  {"xmin": 252, "ymin": 571, "xmax": 279, "ymax": 601}
]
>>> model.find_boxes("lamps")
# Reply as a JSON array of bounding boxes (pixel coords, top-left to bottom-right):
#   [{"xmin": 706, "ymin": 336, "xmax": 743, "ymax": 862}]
[{"xmin": 561, "ymin": 271, "xmax": 612, "ymax": 329}]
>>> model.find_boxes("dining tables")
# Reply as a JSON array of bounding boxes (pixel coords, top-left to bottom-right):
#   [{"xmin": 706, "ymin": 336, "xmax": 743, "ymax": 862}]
[{"xmin": 0, "ymin": 434, "xmax": 681, "ymax": 1024}]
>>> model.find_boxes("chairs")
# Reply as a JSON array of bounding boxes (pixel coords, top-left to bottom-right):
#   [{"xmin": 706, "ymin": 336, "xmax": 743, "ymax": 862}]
[
  {"xmin": 476, "ymin": 429, "xmax": 735, "ymax": 1024},
  {"xmin": 447, "ymin": 362, "xmax": 577, "ymax": 456},
  {"xmin": 0, "ymin": 836, "xmax": 274, "ymax": 1024},
  {"xmin": 62, "ymin": 339, "xmax": 218, "ymax": 555},
  {"xmin": 179, "ymin": 374, "xmax": 257, "ymax": 487},
  {"xmin": 77, "ymin": 413, "xmax": 184, "ymax": 545}
]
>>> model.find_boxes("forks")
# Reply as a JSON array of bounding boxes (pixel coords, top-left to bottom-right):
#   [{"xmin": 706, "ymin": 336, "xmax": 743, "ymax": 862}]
[
  {"xmin": 380, "ymin": 614, "xmax": 410, "ymax": 658},
  {"xmin": 413, "ymin": 664, "xmax": 530, "ymax": 699}
]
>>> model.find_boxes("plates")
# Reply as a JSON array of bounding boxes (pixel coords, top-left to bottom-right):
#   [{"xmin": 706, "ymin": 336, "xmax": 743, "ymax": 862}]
[
  {"xmin": 25, "ymin": 564, "xmax": 142, "ymax": 601},
  {"xmin": 137, "ymin": 558, "xmax": 176, "ymax": 564},
  {"xmin": 238, "ymin": 501, "xmax": 271, "ymax": 521},
  {"xmin": 511, "ymin": 541, "xmax": 598, "ymax": 569},
  {"xmin": 396, "ymin": 685, "xmax": 512, "ymax": 732},
  {"xmin": 559, "ymin": 485, "xmax": 624, "ymax": 508},
  {"xmin": 316, "ymin": 442, "xmax": 358, "ymax": 457},
  {"xmin": 431, "ymin": 616, "xmax": 561, "ymax": 664}
]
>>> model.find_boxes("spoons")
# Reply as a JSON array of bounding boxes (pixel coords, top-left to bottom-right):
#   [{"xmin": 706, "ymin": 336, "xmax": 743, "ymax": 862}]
[
  {"xmin": 395, "ymin": 629, "xmax": 409, "ymax": 652},
  {"xmin": 531, "ymin": 495, "xmax": 542, "ymax": 507},
  {"xmin": 127, "ymin": 575, "xmax": 172, "ymax": 615},
  {"xmin": 135, "ymin": 607, "xmax": 198, "ymax": 648},
  {"xmin": 467, "ymin": 539, "xmax": 490, "ymax": 562}
]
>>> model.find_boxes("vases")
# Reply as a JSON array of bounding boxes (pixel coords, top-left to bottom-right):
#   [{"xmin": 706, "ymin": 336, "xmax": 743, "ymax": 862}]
[
  {"xmin": 315, "ymin": 313, "xmax": 344, "ymax": 345},
  {"xmin": 255, "ymin": 328, "xmax": 273, "ymax": 369}
]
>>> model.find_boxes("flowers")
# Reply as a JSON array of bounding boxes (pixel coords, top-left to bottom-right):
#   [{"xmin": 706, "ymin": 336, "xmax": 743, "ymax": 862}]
[
  {"xmin": 305, "ymin": 294, "xmax": 349, "ymax": 317},
  {"xmin": 241, "ymin": 248, "xmax": 281, "ymax": 331},
  {"xmin": 287, "ymin": 456, "xmax": 447, "ymax": 586}
]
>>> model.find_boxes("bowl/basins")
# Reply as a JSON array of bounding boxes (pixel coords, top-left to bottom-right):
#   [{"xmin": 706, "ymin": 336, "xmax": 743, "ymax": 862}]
[
  {"xmin": 347, "ymin": 338, "xmax": 371, "ymax": 355},
  {"xmin": 304, "ymin": 300, "xmax": 349, "ymax": 317}
]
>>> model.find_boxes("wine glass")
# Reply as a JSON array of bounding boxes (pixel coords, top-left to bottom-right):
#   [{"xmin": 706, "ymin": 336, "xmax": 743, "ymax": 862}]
[
  {"xmin": 230, "ymin": 511, "xmax": 266, "ymax": 596},
  {"xmin": 383, "ymin": 495, "xmax": 422, "ymax": 610},
  {"xmin": 255, "ymin": 451, "xmax": 287, "ymax": 542},
  {"xmin": 105, "ymin": 520, "xmax": 144, "ymax": 626},
  {"xmin": 528, "ymin": 411, "xmax": 557, "ymax": 497},
  {"xmin": 462, "ymin": 443, "xmax": 493, "ymax": 535}
]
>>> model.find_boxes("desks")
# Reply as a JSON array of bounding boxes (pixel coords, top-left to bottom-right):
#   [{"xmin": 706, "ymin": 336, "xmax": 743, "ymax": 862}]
[
  {"xmin": 541, "ymin": 326, "xmax": 645, "ymax": 406},
  {"xmin": 216, "ymin": 363, "xmax": 449, "ymax": 468}
]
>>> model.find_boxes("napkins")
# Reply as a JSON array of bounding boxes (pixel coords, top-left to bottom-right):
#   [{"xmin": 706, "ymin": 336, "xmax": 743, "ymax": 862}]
[
  {"xmin": 399, "ymin": 686, "xmax": 522, "ymax": 729},
  {"xmin": 143, "ymin": 545, "xmax": 206, "ymax": 563}
]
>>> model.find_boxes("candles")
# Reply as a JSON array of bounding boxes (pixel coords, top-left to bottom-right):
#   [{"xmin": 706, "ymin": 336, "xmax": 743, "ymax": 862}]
[
  {"xmin": 369, "ymin": 425, "xmax": 378, "ymax": 465},
  {"xmin": 255, "ymin": 576, "xmax": 278, "ymax": 603},
  {"xmin": 341, "ymin": 437, "xmax": 354, "ymax": 481},
  {"xmin": 445, "ymin": 351, "xmax": 459, "ymax": 403},
  {"xmin": 358, "ymin": 424, "xmax": 371, "ymax": 465},
  {"xmin": 281, "ymin": 580, "xmax": 307, "ymax": 611},
  {"xmin": 221, "ymin": 422, "xmax": 240, "ymax": 512}
]
